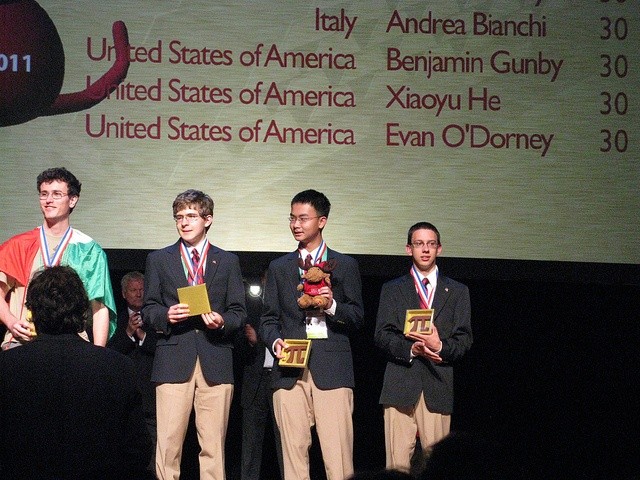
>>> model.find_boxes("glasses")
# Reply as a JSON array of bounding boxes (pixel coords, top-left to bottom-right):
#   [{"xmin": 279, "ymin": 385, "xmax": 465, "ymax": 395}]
[
  {"xmin": 174, "ymin": 214, "xmax": 202, "ymax": 222},
  {"xmin": 288, "ymin": 217, "xmax": 317, "ymax": 223},
  {"xmin": 39, "ymin": 191, "xmax": 69, "ymax": 199},
  {"xmin": 412, "ymin": 241, "xmax": 437, "ymax": 247}
]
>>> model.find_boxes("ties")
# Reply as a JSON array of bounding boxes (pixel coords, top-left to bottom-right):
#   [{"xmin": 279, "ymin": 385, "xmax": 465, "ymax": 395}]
[
  {"xmin": 304, "ymin": 254, "xmax": 312, "ymax": 274},
  {"xmin": 422, "ymin": 278, "xmax": 429, "ymax": 295},
  {"xmin": 188, "ymin": 248, "xmax": 204, "ymax": 286}
]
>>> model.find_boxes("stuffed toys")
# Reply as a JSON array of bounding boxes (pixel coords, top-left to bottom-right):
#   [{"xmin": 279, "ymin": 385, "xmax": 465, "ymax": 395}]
[{"xmin": 296, "ymin": 257, "xmax": 337, "ymax": 310}]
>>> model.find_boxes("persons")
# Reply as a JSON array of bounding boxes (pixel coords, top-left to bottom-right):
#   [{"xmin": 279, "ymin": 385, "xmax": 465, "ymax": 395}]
[
  {"xmin": 0, "ymin": 166, "xmax": 118, "ymax": 352},
  {"xmin": 0, "ymin": 264, "xmax": 154, "ymax": 480},
  {"xmin": 372, "ymin": 222, "xmax": 474, "ymax": 472},
  {"xmin": 139, "ymin": 188, "xmax": 248, "ymax": 480},
  {"xmin": 107, "ymin": 270, "xmax": 156, "ymax": 480},
  {"xmin": 259, "ymin": 188, "xmax": 364, "ymax": 480},
  {"xmin": 239, "ymin": 268, "xmax": 284, "ymax": 480}
]
